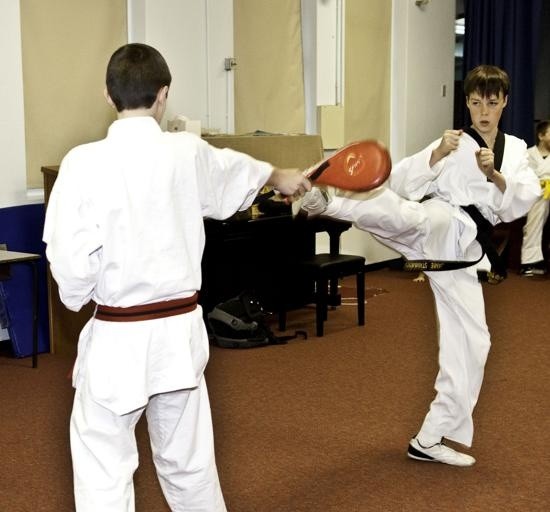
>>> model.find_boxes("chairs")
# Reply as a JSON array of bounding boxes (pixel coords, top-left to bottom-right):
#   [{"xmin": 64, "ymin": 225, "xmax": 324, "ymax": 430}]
[{"xmin": 293, "ymin": 216, "xmax": 365, "ymax": 337}]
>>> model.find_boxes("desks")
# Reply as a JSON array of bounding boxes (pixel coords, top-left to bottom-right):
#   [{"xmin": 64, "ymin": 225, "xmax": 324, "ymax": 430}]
[{"xmin": 0, "ymin": 249, "xmax": 41, "ymax": 367}]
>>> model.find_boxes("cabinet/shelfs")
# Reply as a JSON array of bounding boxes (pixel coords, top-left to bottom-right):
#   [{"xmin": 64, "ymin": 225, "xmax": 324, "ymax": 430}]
[{"xmin": 40, "ymin": 134, "xmax": 324, "ymax": 354}]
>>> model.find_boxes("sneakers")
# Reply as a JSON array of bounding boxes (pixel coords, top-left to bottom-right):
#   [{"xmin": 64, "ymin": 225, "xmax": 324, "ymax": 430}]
[
  {"xmin": 408, "ymin": 434, "xmax": 476, "ymax": 468},
  {"xmin": 274, "ymin": 184, "xmax": 329, "ymax": 220},
  {"xmin": 520, "ymin": 264, "xmax": 544, "ymax": 274}
]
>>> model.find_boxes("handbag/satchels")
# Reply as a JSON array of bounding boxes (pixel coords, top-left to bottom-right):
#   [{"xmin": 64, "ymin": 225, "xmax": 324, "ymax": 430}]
[{"xmin": 208, "ymin": 296, "xmax": 308, "ymax": 349}]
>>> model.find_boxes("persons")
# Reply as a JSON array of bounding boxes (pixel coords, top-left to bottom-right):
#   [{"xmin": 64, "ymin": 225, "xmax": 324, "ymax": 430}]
[
  {"xmin": 41, "ymin": 43, "xmax": 314, "ymax": 510},
  {"xmin": 522, "ymin": 121, "xmax": 550, "ymax": 277},
  {"xmin": 295, "ymin": 63, "xmax": 544, "ymax": 468}
]
